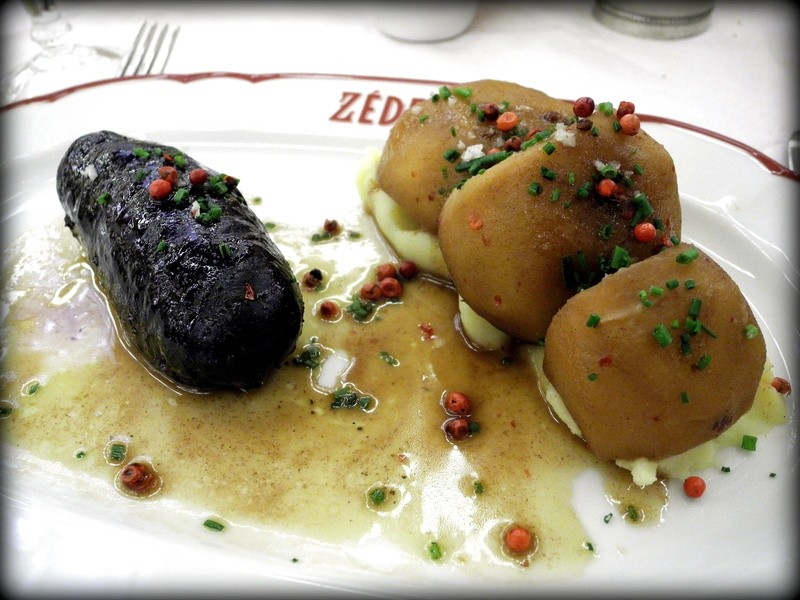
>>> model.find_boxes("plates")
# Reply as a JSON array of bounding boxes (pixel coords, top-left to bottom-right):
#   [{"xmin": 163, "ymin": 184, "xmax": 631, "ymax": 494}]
[{"xmin": 2, "ymin": 74, "xmax": 800, "ymax": 600}]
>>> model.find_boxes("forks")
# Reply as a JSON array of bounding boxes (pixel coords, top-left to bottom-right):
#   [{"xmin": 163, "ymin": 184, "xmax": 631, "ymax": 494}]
[{"xmin": 120, "ymin": 21, "xmax": 182, "ymax": 75}]
